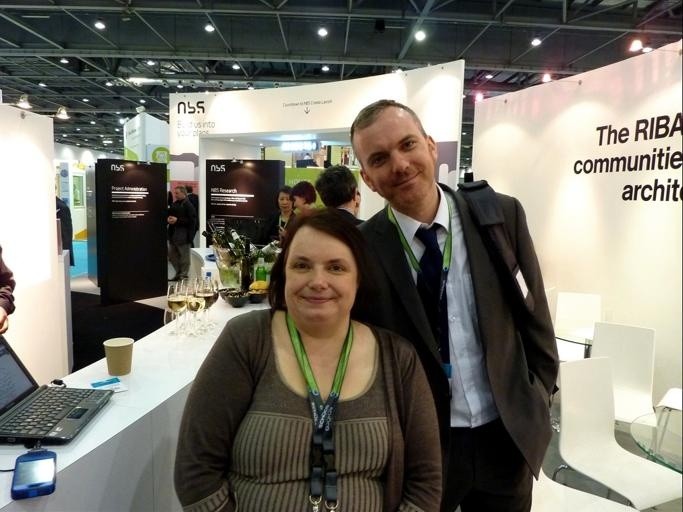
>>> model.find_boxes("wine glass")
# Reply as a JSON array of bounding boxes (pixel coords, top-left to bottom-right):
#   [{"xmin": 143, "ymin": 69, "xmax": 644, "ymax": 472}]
[{"xmin": 162, "ymin": 276, "xmax": 219, "ymax": 338}]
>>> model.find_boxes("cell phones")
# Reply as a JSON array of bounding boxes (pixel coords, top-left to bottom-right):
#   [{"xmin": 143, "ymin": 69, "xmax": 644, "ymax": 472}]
[{"xmin": 11, "ymin": 451, "xmax": 56, "ymax": 500}]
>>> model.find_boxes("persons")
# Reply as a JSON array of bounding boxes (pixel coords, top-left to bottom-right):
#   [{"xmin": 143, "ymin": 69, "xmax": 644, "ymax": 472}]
[
  {"xmin": 315, "ymin": 164, "xmax": 365, "ymax": 226},
  {"xmin": 353, "ymin": 191, "xmax": 360, "ymax": 217},
  {"xmin": 349, "ymin": 99, "xmax": 559, "ymax": 512},
  {"xmin": 54, "ymin": 185, "xmax": 76, "ymax": 267},
  {"xmin": 185, "ymin": 186, "xmax": 198, "ymax": 247},
  {"xmin": 172, "ymin": 206, "xmax": 444, "ymax": 512},
  {"xmin": 0, "ymin": 247, "xmax": 15, "ymax": 334},
  {"xmin": 278, "ymin": 180, "xmax": 318, "ymax": 248},
  {"xmin": 262, "ymin": 185, "xmax": 295, "ymax": 249},
  {"xmin": 166, "ymin": 184, "xmax": 197, "ymax": 282}
]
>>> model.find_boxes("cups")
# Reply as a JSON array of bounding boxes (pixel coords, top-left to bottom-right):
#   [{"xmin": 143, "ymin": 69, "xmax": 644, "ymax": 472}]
[{"xmin": 103, "ymin": 337, "xmax": 133, "ymax": 375}]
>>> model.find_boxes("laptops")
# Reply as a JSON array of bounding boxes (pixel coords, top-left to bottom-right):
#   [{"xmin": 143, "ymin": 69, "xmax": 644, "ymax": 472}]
[{"xmin": 0, "ymin": 334, "xmax": 114, "ymax": 445}]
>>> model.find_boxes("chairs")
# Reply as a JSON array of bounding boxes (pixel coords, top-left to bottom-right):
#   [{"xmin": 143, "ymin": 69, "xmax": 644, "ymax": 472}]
[
  {"xmin": 552, "ymin": 291, "xmax": 599, "ymax": 362},
  {"xmin": 531, "ymin": 445, "xmax": 633, "ymax": 511},
  {"xmin": 589, "ymin": 322, "xmax": 657, "ymax": 443},
  {"xmin": 551, "ymin": 357, "xmax": 682, "ymax": 511},
  {"xmin": 657, "ymin": 388, "xmax": 681, "ymax": 446}
]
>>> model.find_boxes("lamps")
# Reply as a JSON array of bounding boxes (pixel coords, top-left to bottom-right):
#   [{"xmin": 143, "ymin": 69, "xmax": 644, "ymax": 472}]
[
  {"xmin": 541, "ymin": 73, "xmax": 582, "ymax": 88},
  {"xmin": 20, "ymin": 106, "xmax": 71, "ymax": 120},
  {"xmin": 2, "ymin": 94, "xmax": 33, "ymax": 110},
  {"xmin": 627, "ymin": 39, "xmax": 683, "ymax": 56}
]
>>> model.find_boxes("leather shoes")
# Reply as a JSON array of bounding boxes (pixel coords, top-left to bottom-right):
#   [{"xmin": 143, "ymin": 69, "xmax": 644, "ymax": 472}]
[{"xmin": 168, "ymin": 272, "xmax": 188, "ymax": 281}]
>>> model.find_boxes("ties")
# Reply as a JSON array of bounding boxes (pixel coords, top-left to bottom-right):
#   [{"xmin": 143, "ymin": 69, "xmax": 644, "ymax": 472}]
[{"xmin": 415, "ymin": 223, "xmax": 449, "ymax": 383}]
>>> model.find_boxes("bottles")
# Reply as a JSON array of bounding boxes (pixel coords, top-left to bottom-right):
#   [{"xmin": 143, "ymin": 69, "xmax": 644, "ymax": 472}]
[
  {"xmin": 241, "ymin": 235, "xmax": 252, "ymax": 292},
  {"xmin": 200, "ymin": 215, "xmax": 254, "ymax": 256},
  {"xmin": 204, "ymin": 273, "xmax": 213, "ymax": 291},
  {"xmin": 256, "ymin": 256, "xmax": 267, "ymax": 284},
  {"xmin": 247, "ymin": 240, "xmax": 281, "ymax": 263}
]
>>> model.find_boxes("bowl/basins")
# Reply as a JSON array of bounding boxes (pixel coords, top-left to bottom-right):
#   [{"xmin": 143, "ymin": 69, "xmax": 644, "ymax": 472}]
[
  {"xmin": 213, "ymin": 246, "xmax": 243, "ymax": 288},
  {"xmin": 217, "ymin": 288, "xmax": 266, "ymax": 308}
]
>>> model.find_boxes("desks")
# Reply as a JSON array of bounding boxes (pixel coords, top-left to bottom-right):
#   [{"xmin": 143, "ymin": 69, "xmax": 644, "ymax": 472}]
[{"xmin": 633, "ymin": 408, "xmax": 681, "ymax": 471}]
